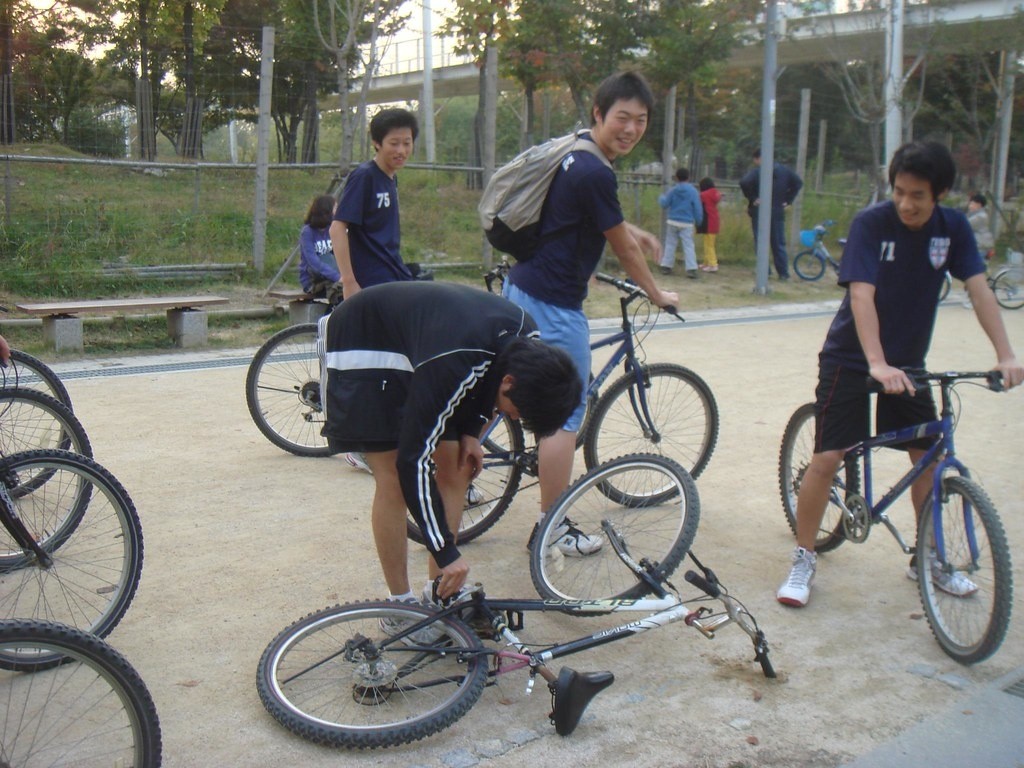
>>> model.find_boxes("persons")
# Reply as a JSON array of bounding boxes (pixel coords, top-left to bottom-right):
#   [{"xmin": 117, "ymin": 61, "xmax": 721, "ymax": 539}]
[
  {"xmin": 775, "ymin": 141, "xmax": 1024, "ymax": 607},
  {"xmin": 316, "ymin": 281, "xmax": 583, "ymax": 647},
  {"xmin": 740, "ymin": 147, "xmax": 803, "ymax": 280},
  {"xmin": 0, "ymin": 333, "xmax": 10, "ymax": 365},
  {"xmin": 503, "ymin": 72, "xmax": 680, "ymax": 557},
  {"xmin": 696, "ymin": 177, "xmax": 721, "ymax": 272},
  {"xmin": 329, "ymin": 108, "xmax": 484, "ymax": 509},
  {"xmin": 659, "ymin": 168, "xmax": 703, "ymax": 279},
  {"xmin": 961, "ymin": 194, "xmax": 994, "ymax": 309},
  {"xmin": 299, "ymin": 195, "xmax": 344, "ymax": 313}
]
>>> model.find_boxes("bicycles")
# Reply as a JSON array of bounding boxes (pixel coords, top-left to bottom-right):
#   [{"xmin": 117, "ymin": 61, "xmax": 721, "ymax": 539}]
[
  {"xmin": 242, "ymin": 261, "xmax": 515, "ymax": 458},
  {"xmin": 0, "ymin": 350, "xmax": 164, "ymax": 768},
  {"xmin": 938, "ymin": 248, "xmax": 1024, "ymax": 311},
  {"xmin": 792, "ymin": 217, "xmax": 851, "ymax": 282},
  {"xmin": 778, "ymin": 370, "xmax": 1013, "ymax": 669},
  {"xmin": 257, "ymin": 450, "xmax": 777, "ymax": 751},
  {"xmin": 405, "ymin": 272, "xmax": 721, "ymax": 547}
]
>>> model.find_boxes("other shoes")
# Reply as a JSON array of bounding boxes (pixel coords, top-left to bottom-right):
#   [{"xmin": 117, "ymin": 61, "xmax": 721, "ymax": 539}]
[
  {"xmin": 661, "ymin": 265, "xmax": 672, "ymax": 274},
  {"xmin": 686, "ymin": 269, "xmax": 697, "ymax": 278}
]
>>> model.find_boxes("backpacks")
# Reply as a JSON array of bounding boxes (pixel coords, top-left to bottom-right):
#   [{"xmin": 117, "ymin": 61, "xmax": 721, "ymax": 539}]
[{"xmin": 477, "ymin": 128, "xmax": 615, "ymax": 255}]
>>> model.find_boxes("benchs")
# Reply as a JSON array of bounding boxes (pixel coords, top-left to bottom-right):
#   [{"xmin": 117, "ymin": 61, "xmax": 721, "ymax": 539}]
[
  {"xmin": 16, "ymin": 296, "xmax": 231, "ymax": 355},
  {"xmin": 269, "ymin": 289, "xmax": 328, "ymax": 337}
]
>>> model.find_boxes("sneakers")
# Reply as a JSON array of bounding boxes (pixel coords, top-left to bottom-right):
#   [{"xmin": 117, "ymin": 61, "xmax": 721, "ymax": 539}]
[
  {"xmin": 345, "ymin": 451, "xmax": 374, "ymax": 473},
  {"xmin": 906, "ymin": 546, "xmax": 980, "ymax": 598},
  {"xmin": 378, "ymin": 598, "xmax": 453, "ymax": 650},
  {"xmin": 776, "ymin": 545, "xmax": 818, "ymax": 607},
  {"xmin": 466, "ymin": 486, "xmax": 484, "ymax": 509},
  {"xmin": 697, "ymin": 263, "xmax": 718, "ymax": 271},
  {"xmin": 526, "ymin": 513, "xmax": 604, "ymax": 558},
  {"xmin": 422, "ymin": 574, "xmax": 484, "ymax": 621}
]
